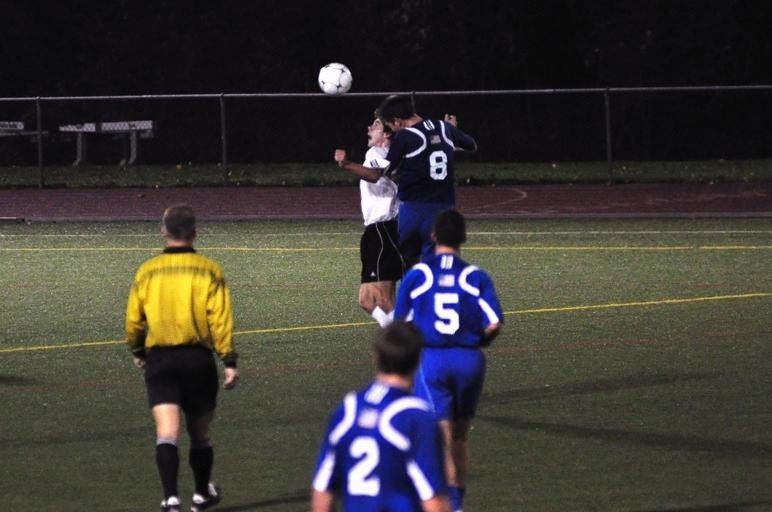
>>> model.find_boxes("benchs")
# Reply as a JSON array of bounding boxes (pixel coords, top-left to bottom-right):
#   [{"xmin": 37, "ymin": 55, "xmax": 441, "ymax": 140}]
[
  {"xmin": 58, "ymin": 120, "xmax": 159, "ymax": 166},
  {"xmin": 0, "ymin": 121, "xmax": 25, "ymax": 133}
]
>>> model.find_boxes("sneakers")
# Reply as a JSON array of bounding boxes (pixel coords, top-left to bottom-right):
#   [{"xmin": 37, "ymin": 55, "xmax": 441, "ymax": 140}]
[
  {"xmin": 160, "ymin": 496, "xmax": 182, "ymax": 512},
  {"xmin": 191, "ymin": 483, "xmax": 222, "ymax": 512}
]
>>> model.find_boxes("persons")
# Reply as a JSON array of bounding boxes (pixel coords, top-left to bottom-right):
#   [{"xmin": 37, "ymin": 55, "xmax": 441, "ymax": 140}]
[
  {"xmin": 335, "ymin": 116, "xmax": 402, "ymax": 328},
  {"xmin": 125, "ymin": 204, "xmax": 239, "ymax": 511},
  {"xmin": 311, "ymin": 321, "xmax": 464, "ymax": 510},
  {"xmin": 393, "ymin": 208, "xmax": 504, "ymax": 508},
  {"xmin": 376, "ymin": 95, "xmax": 478, "ymax": 265}
]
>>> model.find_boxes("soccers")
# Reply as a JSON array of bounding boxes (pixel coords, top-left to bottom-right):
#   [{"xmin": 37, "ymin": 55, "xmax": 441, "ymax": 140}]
[{"xmin": 319, "ymin": 63, "xmax": 353, "ymax": 93}]
